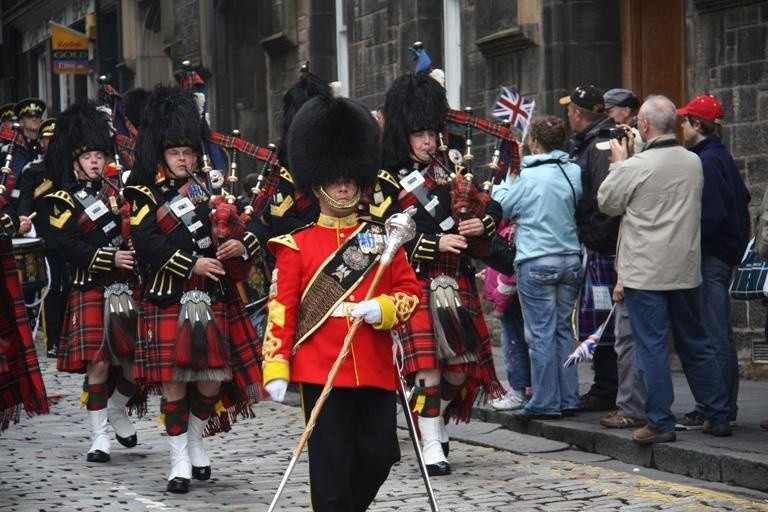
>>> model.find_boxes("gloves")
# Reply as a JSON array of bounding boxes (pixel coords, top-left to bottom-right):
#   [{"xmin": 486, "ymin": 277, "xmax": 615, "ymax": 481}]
[
  {"xmin": 264, "ymin": 380, "xmax": 287, "ymax": 402},
  {"xmin": 351, "ymin": 299, "xmax": 381, "ymax": 324}
]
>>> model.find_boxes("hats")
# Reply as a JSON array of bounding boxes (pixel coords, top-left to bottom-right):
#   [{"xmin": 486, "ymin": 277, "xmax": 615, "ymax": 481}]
[
  {"xmin": 604, "ymin": 89, "xmax": 637, "ymax": 109},
  {"xmin": 280, "ymin": 64, "xmax": 383, "ymax": 197},
  {"xmin": 0, "ymin": 98, "xmax": 55, "ymax": 137},
  {"xmin": 559, "ymin": 81, "xmax": 604, "ymax": 113},
  {"xmin": 675, "ymin": 95, "xmax": 724, "ymax": 124},
  {"xmin": 49, "ymin": 87, "xmax": 202, "ymax": 188},
  {"xmin": 382, "ymin": 75, "xmax": 448, "ymax": 176}
]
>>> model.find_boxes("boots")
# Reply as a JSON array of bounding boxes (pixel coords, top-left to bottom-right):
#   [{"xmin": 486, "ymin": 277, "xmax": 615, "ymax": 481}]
[
  {"xmin": 418, "ymin": 399, "xmax": 450, "ymax": 475},
  {"xmin": 167, "ymin": 433, "xmax": 192, "ymax": 492},
  {"xmin": 187, "ymin": 410, "xmax": 211, "ymax": 480},
  {"xmin": 107, "ymin": 390, "xmax": 137, "ymax": 447},
  {"xmin": 88, "ymin": 407, "xmax": 112, "ymax": 462}
]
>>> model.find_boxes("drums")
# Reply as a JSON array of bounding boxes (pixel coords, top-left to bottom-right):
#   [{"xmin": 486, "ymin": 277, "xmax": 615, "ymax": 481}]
[{"xmin": 11, "ymin": 237, "xmax": 48, "ymax": 288}]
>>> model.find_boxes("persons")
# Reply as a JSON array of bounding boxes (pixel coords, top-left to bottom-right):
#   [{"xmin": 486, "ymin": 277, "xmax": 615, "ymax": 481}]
[
  {"xmin": 0, "ymin": 61, "xmax": 752, "ymax": 494},
  {"xmin": 258, "ymin": 93, "xmax": 425, "ymax": 512}
]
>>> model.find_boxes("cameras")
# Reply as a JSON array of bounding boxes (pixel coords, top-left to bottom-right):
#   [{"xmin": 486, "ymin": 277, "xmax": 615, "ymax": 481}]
[{"xmin": 598, "ymin": 126, "xmax": 629, "ymax": 139}]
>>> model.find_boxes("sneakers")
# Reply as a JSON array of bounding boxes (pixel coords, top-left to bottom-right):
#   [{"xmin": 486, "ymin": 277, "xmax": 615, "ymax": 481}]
[
  {"xmin": 489, "ymin": 386, "xmax": 617, "ymax": 419},
  {"xmin": 601, "ymin": 410, "xmax": 737, "ymax": 443}
]
